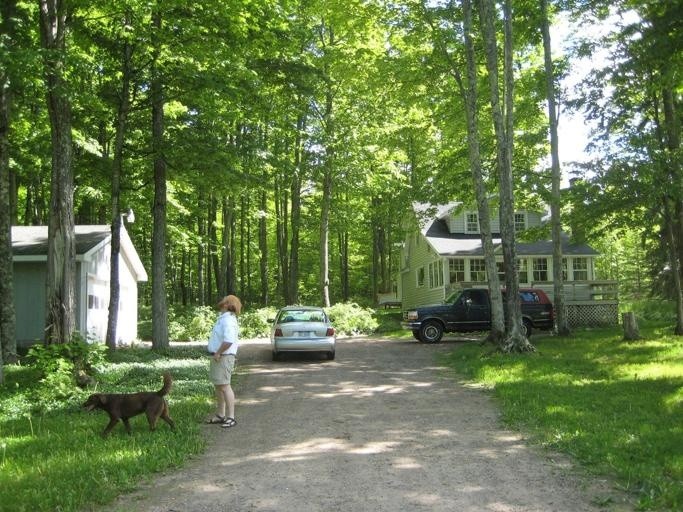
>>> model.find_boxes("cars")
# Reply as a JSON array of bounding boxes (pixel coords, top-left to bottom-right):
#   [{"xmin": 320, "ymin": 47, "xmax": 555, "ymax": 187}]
[
  {"xmin": 267, "ymin": 306, "xmax": 336, "ymax": 361},
  {"xmin": 401, "ymin": 288, "xmax": 553, "ymax": 344}
]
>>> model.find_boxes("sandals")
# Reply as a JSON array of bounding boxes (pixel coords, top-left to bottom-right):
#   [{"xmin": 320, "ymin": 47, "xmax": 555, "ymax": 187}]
[
  {"xmin": 221, "ymin": 417, "xmax": 237, "ymax": 428},
  {"xmin": 205, "ymin": 414, "xmax": 226, "ymax": 424}
]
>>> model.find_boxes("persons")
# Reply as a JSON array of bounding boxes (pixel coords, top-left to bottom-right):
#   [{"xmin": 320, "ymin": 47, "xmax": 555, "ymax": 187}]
[{"xmin": 204, "ymin": 294, "xmax": 243, "ymax": 428}]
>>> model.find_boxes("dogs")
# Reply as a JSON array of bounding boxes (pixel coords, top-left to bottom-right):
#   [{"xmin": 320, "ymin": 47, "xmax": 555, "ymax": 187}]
[{"xmin": 81, "ymin": 371, "xmax": 175, "ymax": 439}]
